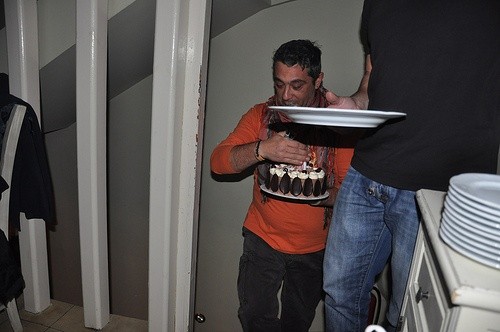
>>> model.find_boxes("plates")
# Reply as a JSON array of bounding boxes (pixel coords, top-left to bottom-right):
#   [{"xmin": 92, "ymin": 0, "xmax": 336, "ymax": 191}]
[
  {"xmin": 438, "ymin": 173, "xmax": 500, "ymax": 270},
  {"xmin": 267, "ymin": 105, "xmax": 407, "ymax": 128},
  {"xmin": 260, "ymin": 184, "xmax": 330, "ymax": 200}
]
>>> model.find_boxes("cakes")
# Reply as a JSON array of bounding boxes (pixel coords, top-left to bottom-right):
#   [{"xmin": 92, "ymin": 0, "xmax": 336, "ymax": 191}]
[{"xmin": 265, "ymin": 162, "xmax": 328, "ymax": 197}]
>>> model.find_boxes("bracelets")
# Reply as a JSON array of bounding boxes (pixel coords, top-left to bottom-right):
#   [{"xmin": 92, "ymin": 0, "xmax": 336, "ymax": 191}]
[{"xmin": 254, "ymin": 140, "xmax": 265, "ymax": 162}]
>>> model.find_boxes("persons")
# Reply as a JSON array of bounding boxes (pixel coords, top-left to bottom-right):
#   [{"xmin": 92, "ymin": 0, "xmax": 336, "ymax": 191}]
[
  {"xmin": 323, "ymin": 0, "xmax": 500, "ymax": 332},
  {"xmin": 211, "ymin": 40, "xmax": 356, "ymax": 332}
]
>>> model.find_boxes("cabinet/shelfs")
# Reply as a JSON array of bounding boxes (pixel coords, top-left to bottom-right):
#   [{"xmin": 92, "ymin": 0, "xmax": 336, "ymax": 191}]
[{"xmin": 398, "ymin": 190, "xmax": 499, "ymax": 332}]
